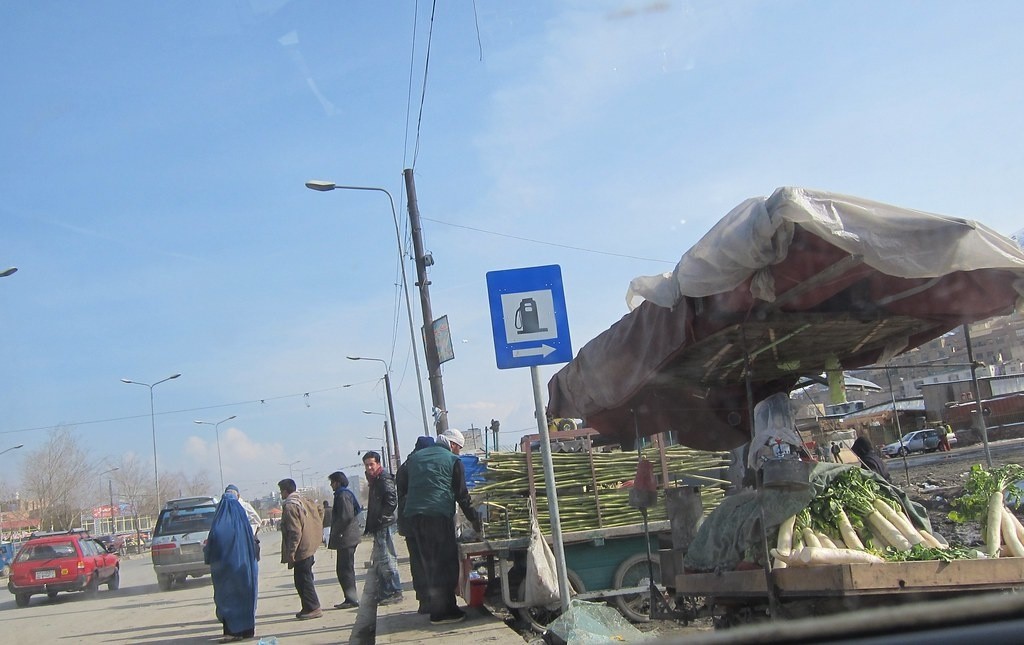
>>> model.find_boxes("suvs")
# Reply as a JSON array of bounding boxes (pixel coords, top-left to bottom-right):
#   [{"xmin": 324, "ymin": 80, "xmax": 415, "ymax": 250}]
[
  {"xmin": 7, "ymin": 527, "xmax": 120, "ymax": 607},
  {"xmin": 150, "ymin": 502, "xmax": 225, "ymax": 590},
  {"xmin": 165, "ymin": 497, "xmax": 219, "ymax": 507}
]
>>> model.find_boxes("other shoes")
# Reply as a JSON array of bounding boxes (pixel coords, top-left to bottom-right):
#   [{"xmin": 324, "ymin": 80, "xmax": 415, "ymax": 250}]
[
  {"xmin": 300, "ymin": 607, "xmax": 323, "ymax": 619},
  {"xmin": 219, "ymin": 633, "xmax": 243, "ymax": 643},
  {"xmin": 296, "ymin": 612, "xmax": 302, "ymax": 618},
  {"xmin": 430, "ymin": 609, "xmax": 466, "ymax": 625},
  {"xmin": 419, "ymin": 605, "xmax": 430, "ymax": 613},
  {"xmin": 245, "ymin": 631, "xmax": 254, "ymax": 639},
  {"xmin": 379, "ymin": 592, "xmax": 404, "ymax": 606},
  {"xmin": 334, "ymin": 599, "xmax": 360, "ymax": 609}
]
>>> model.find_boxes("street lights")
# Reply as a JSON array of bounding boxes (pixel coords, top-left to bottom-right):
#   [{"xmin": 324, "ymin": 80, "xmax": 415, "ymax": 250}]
[
  {"xmin": 100, "ymin": 465, "xmax": 120, "ymax": 534},
  {"xmin": 292, "ymin": 467, "xmax": 310, "ymax": 487},
  {"xmin": 346, "ymin": 354, "xmax": 402, "ymax": 471},
  {"xmin": 363, "ymin": 411, "xmax": 394, "ymax": 476},
  {"xmin": 120, "ymin": 372, "xmax": 183, "ymax": 520},
  {"xmin": 281, "ymin": 459, "xmax": 300, "ymax": 480},
  {"xmin": 358, "ymin": 446, "xmax": 386, "ymax": 469},
  {"xmin": 301, "ymin": 176, "xmax": 431, "ymax": 442},
  {"xmin": 366, "ymin": 436, "xmax": 389, "ymax": 472},
  {"xmin": 192, "ymin": 414, "xmax": 238, "ymax": 497}
]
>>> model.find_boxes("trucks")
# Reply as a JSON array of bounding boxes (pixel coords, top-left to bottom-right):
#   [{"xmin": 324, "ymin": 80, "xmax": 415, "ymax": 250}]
[{"xmin": 0, "ymin": 543, "xmax": 20, "ymax": 577}]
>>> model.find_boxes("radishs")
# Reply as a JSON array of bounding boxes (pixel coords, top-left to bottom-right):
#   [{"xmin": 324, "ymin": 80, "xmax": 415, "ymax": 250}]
[{"xmin": 770, "ymin": 490, "xmax": 1024, "ymax": 569}]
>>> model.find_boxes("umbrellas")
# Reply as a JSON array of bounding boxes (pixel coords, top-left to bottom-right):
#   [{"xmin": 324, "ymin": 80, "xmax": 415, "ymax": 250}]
[{"xmin": 266, "ymin": 508, "xmax": 282, "ymax": 518}]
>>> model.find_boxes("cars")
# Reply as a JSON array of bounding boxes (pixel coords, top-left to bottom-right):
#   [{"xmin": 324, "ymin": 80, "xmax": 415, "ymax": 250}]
[
  {"xmin": 883, "ymin": 427, "xmax": 958, "ymax": 457},
  {"xmin": 97, "ymin": 528, "xmax": 154, "ymax": 555}
]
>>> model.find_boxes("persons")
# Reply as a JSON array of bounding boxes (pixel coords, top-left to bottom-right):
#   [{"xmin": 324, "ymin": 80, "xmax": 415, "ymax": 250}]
[
  {"xmin": 396, "ymin": 436, "xmax": 437, "ymax": 615},
  {"xmin": 225, "ymin": 484, "xmax": 263, "ymax": 628},
  {"xmin": 937, "ymin": 420, "xmax": 953, "ymax": 451},
  {"xmin": 277, "ymin": 478, "xmax": 324, "ymax": 621},
  {"xmin": 363, "ymin": 451, "xmax": 404, "ymax": 606},
  {"xmin": 322, "ymin": 500, "xmax": 333, "ymax": 547},
  {"xmin": 202, "ymin": 493, "xmax": 259, "ymax": 640},
  {"xmin": 815, "ymin": 442, "xmax": 844, "ymax": 465},
  {"xmin": 850, "ymin": 436, "xmax": 890, "ymax": 481},
  {"xmin": 396, "ymin": 428, "xmax": 483, "ymax": 625},
  {"xmin": 327, "ymin": 471, "xmax": 362, "ymax": 610}
]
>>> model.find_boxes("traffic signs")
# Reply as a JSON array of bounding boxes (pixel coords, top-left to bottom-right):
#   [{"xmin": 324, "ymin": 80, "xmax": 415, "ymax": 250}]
[{"xmin": 485, "ymin": 265, "xmax": 576, "ymax": 371}]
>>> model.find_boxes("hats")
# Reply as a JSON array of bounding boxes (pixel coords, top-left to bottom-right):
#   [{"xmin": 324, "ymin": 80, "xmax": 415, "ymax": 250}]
[
  {"xmin": 442, "ymin": 428, "xmax": 465, "ymax": 448},
  {"xmin": 416, "ymin": 436, "xmax": 435, "ymax": 450},
  {"xmin": 224, "ymin": 485, "xmax": 238, "ymax": 493}
]
